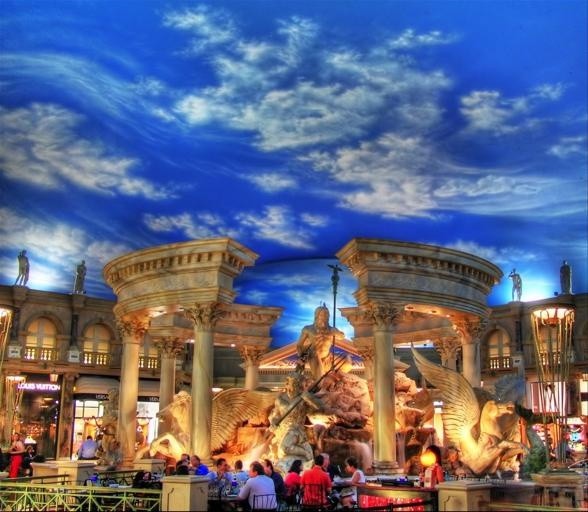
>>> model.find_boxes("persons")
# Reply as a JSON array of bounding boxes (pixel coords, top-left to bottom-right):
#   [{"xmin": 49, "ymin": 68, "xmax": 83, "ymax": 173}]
[
  {"xmin": 271, "ymin": 376, "xmax": 313, "ymax": 462},
  {"xmin": 508, "ymin": 268, "xmax": 522, "ymax": 301},
  {"xmin": 560, "ymin": 260, "xmax": 573, "ymax": 295},
  {"xmin": 0, "ymin": 432, "xmax": 446, "ymax": 512},
  {"xmin": 14, "ymin": 250, "xmax": 28, "ymax": 288},
  {"xmin": 74, "ymin": 260, "xmax": 87, "ymax": 291},
  {"xmin": 295, "ymin": 303, "xmax": 336, "ymax": 374}
]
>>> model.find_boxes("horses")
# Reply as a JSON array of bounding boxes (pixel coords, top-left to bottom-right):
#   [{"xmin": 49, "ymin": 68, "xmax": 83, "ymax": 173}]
[
  {"xmin": 458, "ymin": 400, "xmax": 532, "ymax": 475},
  {"xmin": 134, "ymin": 390, "xmax": 192, "ymax": 463}
]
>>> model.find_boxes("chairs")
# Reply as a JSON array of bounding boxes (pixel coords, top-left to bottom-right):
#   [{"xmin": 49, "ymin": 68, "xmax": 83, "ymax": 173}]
[{"xmin": 253, "ymin": 484, "xmax": 323, "ymax": 512}]
